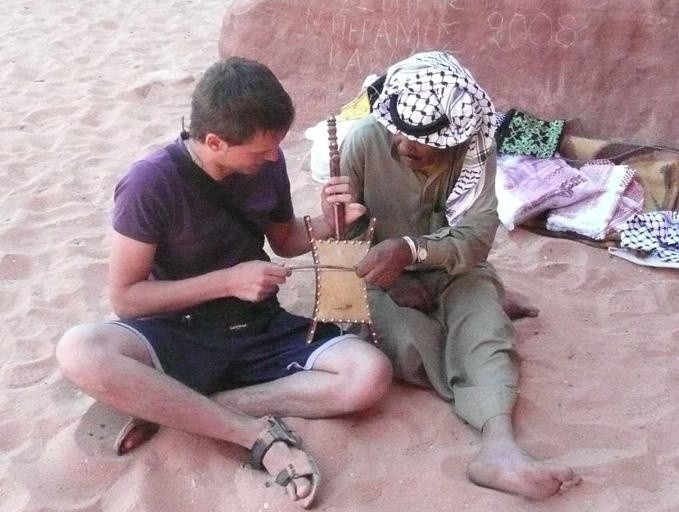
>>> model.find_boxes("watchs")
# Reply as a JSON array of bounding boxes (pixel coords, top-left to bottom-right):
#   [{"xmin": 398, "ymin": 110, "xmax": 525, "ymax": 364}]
[{"xmin": 411, "ymin": 236, "xmax": 428, "ymax": 262}]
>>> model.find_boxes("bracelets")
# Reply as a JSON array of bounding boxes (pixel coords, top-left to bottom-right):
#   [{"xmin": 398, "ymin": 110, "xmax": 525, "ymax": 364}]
[{"xmin": 402, "ymin": 234, "xmax": 417, "ymax": 267}]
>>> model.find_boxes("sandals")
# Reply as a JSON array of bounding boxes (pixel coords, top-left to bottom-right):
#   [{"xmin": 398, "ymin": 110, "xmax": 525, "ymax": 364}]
[
  {"xmin": 116, "ymin": 417, "xmax": 160, "ymax": 456},
  {"xmin": 250, "ymin": 414, "xmax": 322, "ymax": 509}
]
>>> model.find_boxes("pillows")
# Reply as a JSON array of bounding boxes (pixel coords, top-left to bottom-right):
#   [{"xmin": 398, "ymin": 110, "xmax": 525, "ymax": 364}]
[{"xmin": 486, "ymin": 153, "xmax": 648, "ymax": 247}]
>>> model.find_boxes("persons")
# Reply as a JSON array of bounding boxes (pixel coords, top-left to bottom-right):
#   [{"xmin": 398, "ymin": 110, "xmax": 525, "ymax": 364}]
[
  {"xmin": 55, "ymin": 57, "xmax": 397, "ymax": 512},
  {"xmin": 337, "ymin": 49, "xmax": 584, "ymax": 500}
]
